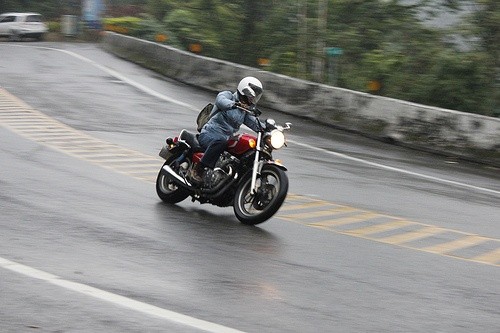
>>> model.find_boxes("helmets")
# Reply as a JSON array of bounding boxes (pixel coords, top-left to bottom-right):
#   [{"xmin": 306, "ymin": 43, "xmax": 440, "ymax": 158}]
[{"xmin": 237, "ymin": 77, "xmax": 264, "ymax": 104}]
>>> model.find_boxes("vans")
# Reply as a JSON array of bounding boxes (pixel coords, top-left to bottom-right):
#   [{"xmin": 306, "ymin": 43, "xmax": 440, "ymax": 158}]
[{"xmin": 1, "ymin": 12, "xmax": 48, "ymax": 42}]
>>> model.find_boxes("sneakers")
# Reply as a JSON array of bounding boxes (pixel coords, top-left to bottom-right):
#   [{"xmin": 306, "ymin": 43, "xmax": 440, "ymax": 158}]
[{"xmin": 185, "ymin": 162, "xmax": 204, "ymax": 185}]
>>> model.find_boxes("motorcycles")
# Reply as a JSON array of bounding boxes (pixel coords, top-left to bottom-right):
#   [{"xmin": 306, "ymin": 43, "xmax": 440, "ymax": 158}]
[{"xmin": 156, "ymin": 103, "xmax": 293, "ymax": 225}]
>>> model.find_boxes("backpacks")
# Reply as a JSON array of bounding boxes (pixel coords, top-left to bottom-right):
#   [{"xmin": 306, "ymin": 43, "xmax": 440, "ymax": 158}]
[{"xmin": 197, "ymin": 103, "xmax": 221, "ymax": 133}]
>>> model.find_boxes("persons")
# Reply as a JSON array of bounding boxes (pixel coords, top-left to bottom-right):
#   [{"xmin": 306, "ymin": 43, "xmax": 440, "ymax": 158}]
[{"xmin": 189, "ymin": 76, "xmax": 268, "ymax": 185}]
